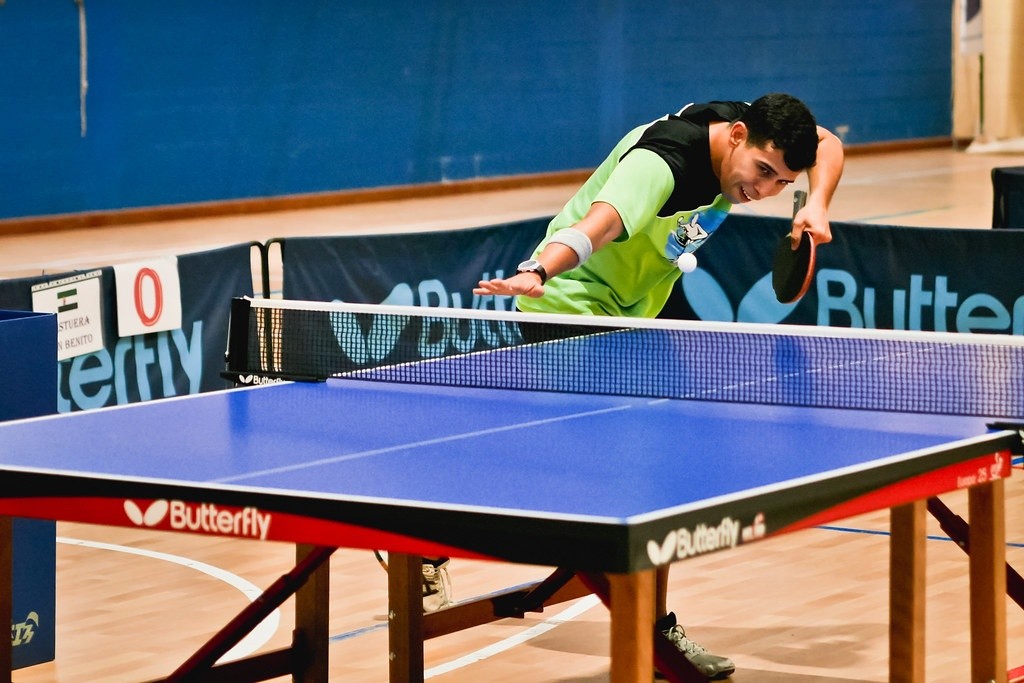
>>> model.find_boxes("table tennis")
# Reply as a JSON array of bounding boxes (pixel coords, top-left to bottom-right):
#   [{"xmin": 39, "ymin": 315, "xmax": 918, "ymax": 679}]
[{"xmin": 677, "ymin": 253, "xmax": 697, "ymax": 273}]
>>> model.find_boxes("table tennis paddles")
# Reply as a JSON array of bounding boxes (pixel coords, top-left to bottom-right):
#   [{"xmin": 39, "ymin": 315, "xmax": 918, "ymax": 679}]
[{"xmin": 771, "ymin": 189, "xmax": 817, "ymax": 305}]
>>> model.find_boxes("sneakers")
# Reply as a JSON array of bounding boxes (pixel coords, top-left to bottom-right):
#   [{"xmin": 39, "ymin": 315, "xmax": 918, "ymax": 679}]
[
  {"xmin": 373, "ymin": 551, "xmax": 456, "ymax": 613},
  {"xmin": 655, "ymin": 612, "xmax": 735, "ymax": 679}
]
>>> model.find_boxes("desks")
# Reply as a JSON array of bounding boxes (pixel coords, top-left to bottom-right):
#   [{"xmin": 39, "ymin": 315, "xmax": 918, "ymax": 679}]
[{"xmin": 0, "ymin": 297, "xmax": 1024, "ymax": 683}]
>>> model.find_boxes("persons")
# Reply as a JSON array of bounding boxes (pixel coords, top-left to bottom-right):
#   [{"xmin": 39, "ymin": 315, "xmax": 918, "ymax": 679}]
[{"xmin": 372, "ymin": 93, "xmax": 846, "ymax": 683}]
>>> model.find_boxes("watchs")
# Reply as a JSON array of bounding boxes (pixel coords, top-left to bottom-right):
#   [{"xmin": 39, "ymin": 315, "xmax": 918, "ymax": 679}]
[{"xmin": 515, "ymin": 260, "xmax": 547, "ymax": 286}]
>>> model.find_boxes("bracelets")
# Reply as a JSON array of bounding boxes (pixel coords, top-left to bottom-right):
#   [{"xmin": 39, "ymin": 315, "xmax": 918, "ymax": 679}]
[{"xmin": 544, "ymin": 227, "xmax": 593, "ymax": 270}]
